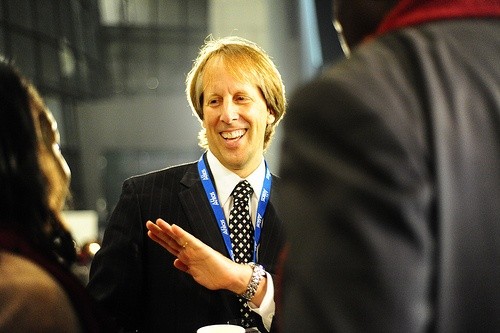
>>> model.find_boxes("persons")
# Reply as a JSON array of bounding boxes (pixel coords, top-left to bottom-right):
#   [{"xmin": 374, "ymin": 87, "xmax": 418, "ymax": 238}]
[
  {"xmin": 87, "ymin": 33, "xmax": 287, "ymax": 333},
  {"xmin": 277, "ymin": 1, "xmax": 500, "ymax": 333},
  {"xmin": 0, "ymin": 55, "xmax": 88, "ymax": 333}
]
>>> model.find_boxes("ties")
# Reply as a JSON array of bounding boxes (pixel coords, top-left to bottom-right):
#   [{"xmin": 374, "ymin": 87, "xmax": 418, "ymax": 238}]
[{"xmin": 229, "ymin": 179, "xmax": 254, "ymax": 333}]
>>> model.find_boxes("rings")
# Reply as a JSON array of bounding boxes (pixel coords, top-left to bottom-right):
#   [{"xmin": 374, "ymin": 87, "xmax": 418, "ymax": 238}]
[{"xmin": 183, "ymin": 241, "xmax": 190, "ymax": 248}]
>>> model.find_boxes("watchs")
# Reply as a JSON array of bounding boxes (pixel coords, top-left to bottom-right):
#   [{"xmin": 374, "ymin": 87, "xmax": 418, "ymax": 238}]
[{"xmin": 240, "ymin": 264, "xmax": 266, "ymax": 302}]
